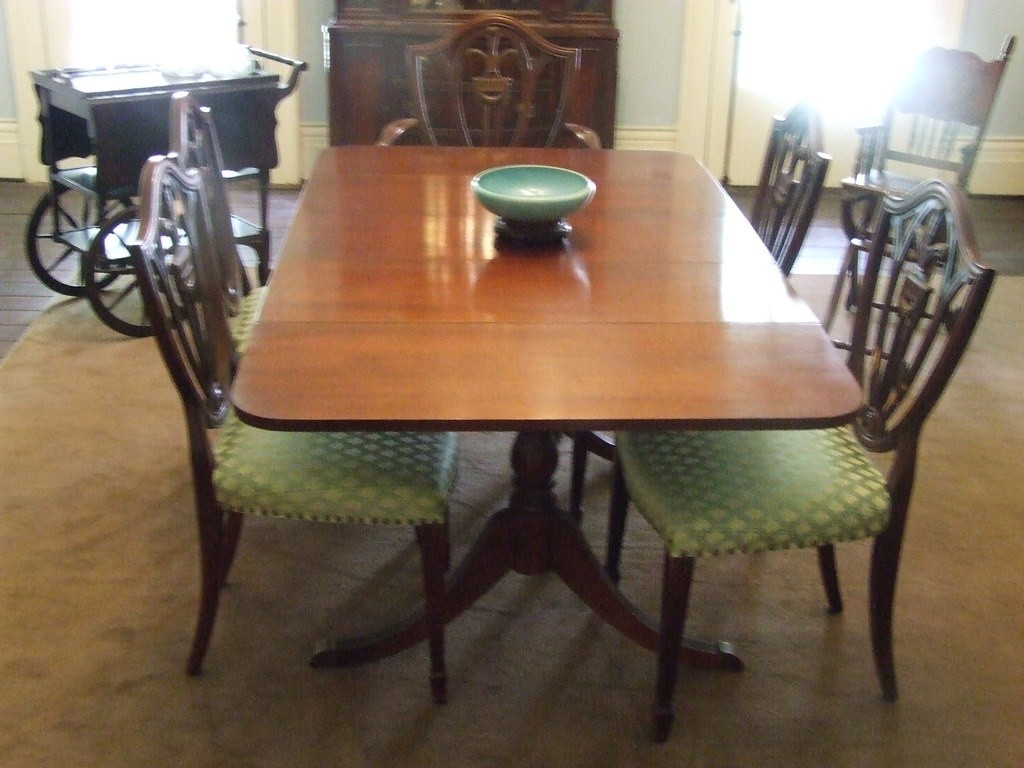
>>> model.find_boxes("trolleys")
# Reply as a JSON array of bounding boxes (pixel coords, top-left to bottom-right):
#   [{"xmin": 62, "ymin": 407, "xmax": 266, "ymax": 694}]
[{"xmin": 24, "ymin": 45, "xmax": 303, "ymax": 338}]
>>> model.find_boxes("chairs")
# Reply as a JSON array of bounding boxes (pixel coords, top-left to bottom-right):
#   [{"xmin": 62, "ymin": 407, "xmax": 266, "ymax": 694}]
[
  {"xmin": 848, "ymin": 32, "xmax": 1016, "ymax": 325},
  {"xmin": 125, "ymin": 154, "xmax": 459, "ymax": 711},
  {"xmin": 377, "ymin": 13, "xmax": 601, "ymax": 150},
  {"xmin": 166, "ymin": 90, "xmax": 271, "ymax": 363},
  {"xmin": 566, "ymin": 104, "xmax": 832, "ymax": 524},
  {"xmin": 604, "ymin": 178, "xmax": 998, "ymax": 746}
]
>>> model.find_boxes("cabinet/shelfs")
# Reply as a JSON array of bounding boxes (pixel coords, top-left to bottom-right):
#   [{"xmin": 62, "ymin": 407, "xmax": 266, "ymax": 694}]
[
  {"xmin": 25, "ymin": 45, "xmax": 308, "ymax": 343},
  {"xmin": 323, "ymin": 0, "xmax": 621, "ymax": 147}
]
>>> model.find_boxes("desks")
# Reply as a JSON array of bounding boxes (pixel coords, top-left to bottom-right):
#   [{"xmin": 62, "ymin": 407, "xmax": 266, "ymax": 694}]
[{"xmin": 229, "ymin": 147, "xmax": 864, "ymax": 674}]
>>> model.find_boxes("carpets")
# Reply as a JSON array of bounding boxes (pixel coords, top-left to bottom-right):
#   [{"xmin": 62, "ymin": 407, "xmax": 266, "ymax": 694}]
[{"xmin": 0, "ymin": 273, "xmax": 1024, "ymax": 768}]
